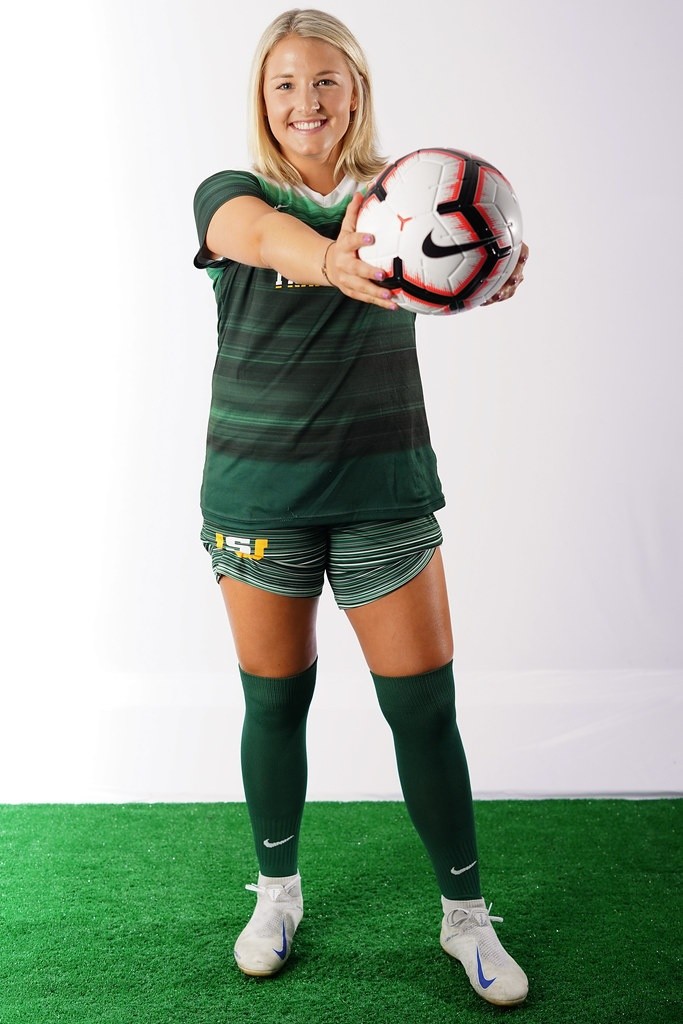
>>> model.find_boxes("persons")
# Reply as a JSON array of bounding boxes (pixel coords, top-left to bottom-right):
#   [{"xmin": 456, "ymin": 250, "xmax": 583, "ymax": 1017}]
[{"xmin": 193, "ymin": 8, "xmax": 528, "ymax": 1007}]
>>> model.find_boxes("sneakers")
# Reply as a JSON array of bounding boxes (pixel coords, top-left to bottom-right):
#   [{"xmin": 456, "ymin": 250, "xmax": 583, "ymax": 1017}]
[
  {"xmin": 440, "ymin": 908, "xmax": 530, "ymax": 1005},
  {"xmin": 234, "ymin": 875, "xmax": 305, "ymax": 976}
]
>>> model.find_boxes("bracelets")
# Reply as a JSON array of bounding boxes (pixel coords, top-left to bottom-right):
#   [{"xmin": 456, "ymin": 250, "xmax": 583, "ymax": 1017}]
[{"xmin": 320, "ymin": 241, "xmax": 337, "ymax": 287}]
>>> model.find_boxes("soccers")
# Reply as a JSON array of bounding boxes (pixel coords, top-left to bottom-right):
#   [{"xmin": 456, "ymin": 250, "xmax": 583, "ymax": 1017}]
[{"xmin": 355, "ymin": 145, "xmax": 524, "ymax": 317}]
